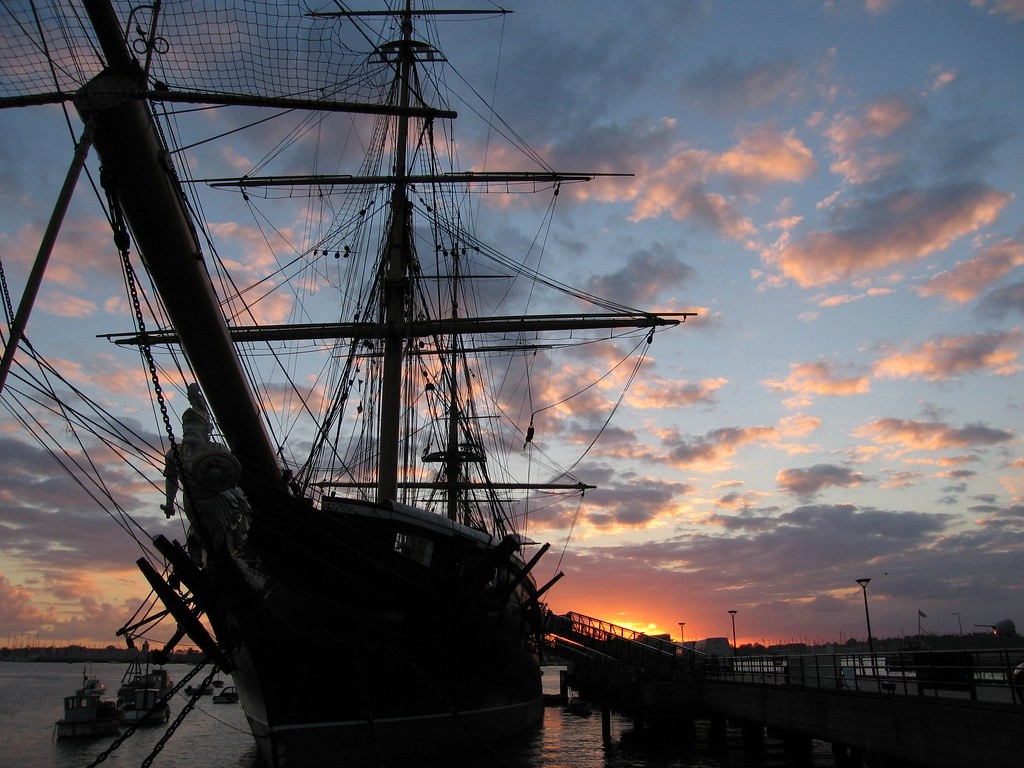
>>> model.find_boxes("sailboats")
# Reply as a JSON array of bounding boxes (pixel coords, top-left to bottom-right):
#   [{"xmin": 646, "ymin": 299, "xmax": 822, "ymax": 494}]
[{"xmin": 1, "ymin": 1, "xmax": 695, "ymax": 768}]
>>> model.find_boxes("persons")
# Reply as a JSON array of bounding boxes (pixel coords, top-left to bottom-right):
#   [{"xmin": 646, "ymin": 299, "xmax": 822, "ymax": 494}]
[{"xmin": 162, "ymin": 383, "xmax": 268, "ymax": 592}]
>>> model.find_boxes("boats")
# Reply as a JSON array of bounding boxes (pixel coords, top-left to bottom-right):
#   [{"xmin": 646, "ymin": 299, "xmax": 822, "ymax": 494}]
[
  {"xmin": 213, "ymin": 686, "xmax": 239, "ymax": 703},
  {"xmin": 117, "ymin": 637, "xmax": 172, "ymax": 726},
  {"xmin": 52, "ymin": 663, "xmax": 120, "ymax": 750},
  {"xmin": 184, "ymin": 682, "xmax": 215, "ymax": 695}
]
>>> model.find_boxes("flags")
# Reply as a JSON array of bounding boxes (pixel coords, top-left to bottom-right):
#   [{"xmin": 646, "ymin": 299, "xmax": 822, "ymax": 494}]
[{"xmin": 920, "ymin": 611, "xmax": 926, "ymax": 616}]
